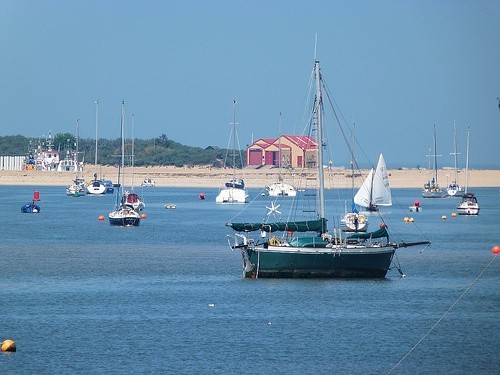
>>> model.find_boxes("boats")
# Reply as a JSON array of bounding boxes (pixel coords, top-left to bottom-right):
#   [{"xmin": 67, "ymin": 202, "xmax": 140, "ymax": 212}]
[
  {"xmin": 407, "ymin": 203, "xmax": 423, "ymax": 212},
  {"xmin": 21, "ymin": 203, "xmax": 41, "ymax": 213}
]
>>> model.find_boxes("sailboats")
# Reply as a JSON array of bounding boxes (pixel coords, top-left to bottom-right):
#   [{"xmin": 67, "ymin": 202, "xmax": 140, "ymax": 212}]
[
  {"xmin": 457, "ymin": 127, "xmax": 480, "ymax": 215},
  {"xmin": 423, "ymin": 126, "xmax": 445, "ymax": 198},
  {"xmin": 224, "ymin": 34, "xmax": 395, "ymax": 279},
  {"xmin": 268, "ymin": 113, "xmax": 297, "ymax": 196},
  {"xmin": 216, "ymin": 100, "xmax": 249, "ymax": 204},
  {"xmin": 67, "ymin": 97, "xmax": 155, "ymax": 226},
  {"xmin": 446, "ymin": 121, "xmax": 464, "ymax": 196}
]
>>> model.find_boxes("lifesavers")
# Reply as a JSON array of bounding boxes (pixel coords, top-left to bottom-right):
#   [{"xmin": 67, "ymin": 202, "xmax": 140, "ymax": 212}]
[
  {"xmin": 269, "ymin": 238, "xmax": 280, "ymax": 246},
  {"xmin": 358, "ymin": 215, "xmax": 365, "ymax": 222}
]
